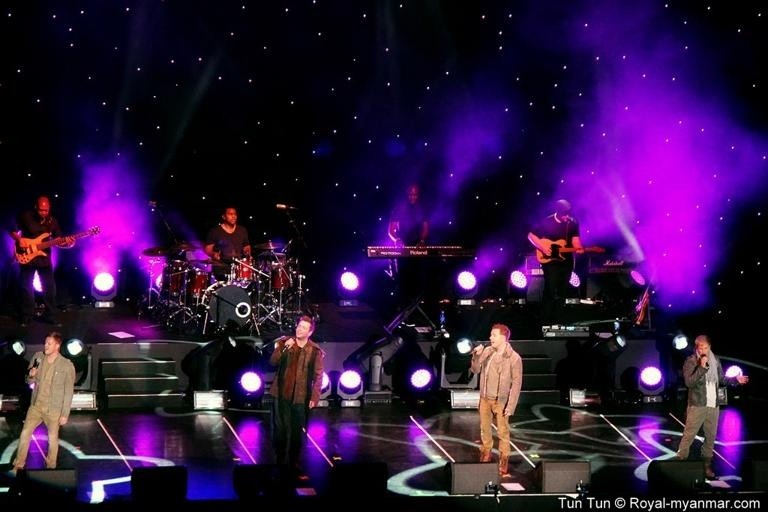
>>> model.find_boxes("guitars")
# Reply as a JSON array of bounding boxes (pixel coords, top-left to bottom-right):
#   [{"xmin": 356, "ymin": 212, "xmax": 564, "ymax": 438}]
[
  {"xmin": 632, "ymin": 288, "xmax": 650, "ymax": 326},
  {"xmin": 14, "ymin": 224, "xmax": 100, "ymax": 264},
  {"xmin": 533, "ymin": 236, "xmax": 604, "ymax": 264}
]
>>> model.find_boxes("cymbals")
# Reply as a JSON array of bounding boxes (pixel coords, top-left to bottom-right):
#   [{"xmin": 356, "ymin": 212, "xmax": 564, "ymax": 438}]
[
  {"xmin": 177, "ymin": 243, "xmax": 199, "ymax": 252},
  {"xmin": 256, "ymin": 242, "xmax": 279, "ymax": 250},
  {"xmin": 143, "ymin": 246, "xmax": 167, "ymax": 257}
]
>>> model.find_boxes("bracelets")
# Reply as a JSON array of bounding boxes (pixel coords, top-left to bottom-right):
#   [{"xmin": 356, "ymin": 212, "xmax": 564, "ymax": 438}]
[{"xmin": 419, "ymin": 239, "xmax": 425, "ymax": 243}]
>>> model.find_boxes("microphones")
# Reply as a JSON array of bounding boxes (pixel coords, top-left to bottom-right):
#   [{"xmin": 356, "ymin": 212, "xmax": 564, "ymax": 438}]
[
  {"xmin": 700, "ymin": 354, "xmax": 709, "ymax": 366},
  {"xmin": 281, "ymin": 337, "xmax": 295, "ymax": 352},
  {"xmin": 469, "ymin": 341, "xmax": 489, "ymax": 355},
  {"xmin": 35, "ymin": 358, "xmax": 41, "ymax": 368}
]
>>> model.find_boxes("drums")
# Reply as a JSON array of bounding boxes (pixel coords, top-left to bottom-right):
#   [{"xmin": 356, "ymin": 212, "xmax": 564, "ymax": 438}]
[
  {"xmin": 272, "ymin": 261, "xmax": 293, "ymax": 289},
  {"xmin": 191, "ymin": 273, "xmax": 210, "ymax": 297},
  {"xmin": 237, "ymin": 258, "xmax": 255, "ymax": 282},
  {"xmin": 201, "ymin": 281, "xmax": 252, "ymax": 331},
  {"xmin": 158, "ymin": 264, "xmax": 194, "ymax": 302}
]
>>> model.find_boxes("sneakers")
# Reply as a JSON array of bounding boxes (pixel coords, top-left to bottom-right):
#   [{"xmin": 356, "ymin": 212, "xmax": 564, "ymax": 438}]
[
  {"xmin": 704, "ymin": 467, "xmax": 719, "ymax": 482},
  {"xmin": 479, "ymin": 453, "xmax": 493, "ymax": 463},
  {"xmin": 47, "ymin": 320, "xmax": 62, "ymax": 329},
  {"xmin": 499, "ymin": 464, "xmax": 509, "ymax": 476}
]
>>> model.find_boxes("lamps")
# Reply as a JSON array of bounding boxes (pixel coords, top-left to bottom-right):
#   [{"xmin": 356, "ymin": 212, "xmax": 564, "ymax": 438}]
[
  {"xmin": 57, "ymin": 323, "xmax": 91, "ymax": 389},
  {"xmin": 314, "ymin": 370, "xmax": 334, "ymax": 410},
  {"xmin": 453, "ymin": 270, "xmax": 481, "ymax": 307},
  {"xmin": 88, "ymin": 267, "xmax": 118, "ymax": 308},
  {"xmin": 336, "ymin": 267, "xmax": 359, "ymax": 310},
  {"xmin": 237, "ymin": 369, "xmax": 265, "ymax": 407},
  {"xmin": 636, "ymin": 364, "xmax": 667, "ymax": 404},
  {"xmin": 504, "ymin": 269, "xmax": 528, "ymax": 306},
  {"xmin": 439, "ymin": 337, "xmax": 481, "ymax": 391},
  {"xmin": 333, "ymin": 365, "xmax": 367, "ymax": 408}
]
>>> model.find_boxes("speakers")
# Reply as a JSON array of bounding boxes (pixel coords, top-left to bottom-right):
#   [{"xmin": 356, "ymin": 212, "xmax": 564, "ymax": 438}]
[
  {"xmin": 232, "ymin": 464, "xmax": 294, "ymax": 497},
  {"xmin": 326, "ymin": 461, "xmax": 387, "ymax": 497},
  {"xmin": 527, "ymin": 460, "xmax": 590, "ymax": 493},
  {"xmin": 131, "ymin": 466, "xmax": 188, "ymax": 500},
  {"xmin": 440, "ymin": 462, "xmax": 499, "ymax": 495},
  {"xmin": 14, "ymin": 468, "xmax": 78, "ymax": 501},
  {"xmin": 647, "ymin": 460, "xmax": 705, "ymax": 497}
]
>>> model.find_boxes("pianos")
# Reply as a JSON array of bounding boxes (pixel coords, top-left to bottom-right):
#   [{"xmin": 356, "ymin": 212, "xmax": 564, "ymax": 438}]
[{"xmin": 366, "ymin": 245, "xmax": 461, "ymax": 259}]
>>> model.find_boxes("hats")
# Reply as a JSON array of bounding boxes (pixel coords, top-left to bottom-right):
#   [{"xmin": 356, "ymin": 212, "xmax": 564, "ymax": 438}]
[{"xmin": 553, "ymin": 199, "xmax": 572, "ymax": 213}]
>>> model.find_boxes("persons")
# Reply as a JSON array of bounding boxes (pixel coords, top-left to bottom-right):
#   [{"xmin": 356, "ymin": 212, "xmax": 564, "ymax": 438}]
[
  {"xmin": 13, "ymin": 332, "xmax": 77, "ymax": 469},
  {"xmin": 12, "ymin": 195, "xmax": 62, "ymax": 329},
  {"xmin": 678, "ymin": 334, "xmax": 749, "ymax": 480},
  {"xmin": 528, "ymin": 198, "xmax": 584, "ymax": 307},
  {"xmin": 269, "ymin": 316, "xmax": 327, "ymax": 465},
  {"xmin": 387, "ymin": 182, "xmax": 431, "ymax": 268},
  {"xmin": 205, "ymin": 207, "xmax": 261, "ymax": 318},
  {"xmin": 469, "ymin": 322, "xmax": 523, "ymax": 477}
]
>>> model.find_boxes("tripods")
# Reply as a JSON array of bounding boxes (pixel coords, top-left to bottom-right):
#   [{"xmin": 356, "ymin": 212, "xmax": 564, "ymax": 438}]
[{"xmin": 138, "ymin": 260, "xmax": 319, "ymax": 335}]
